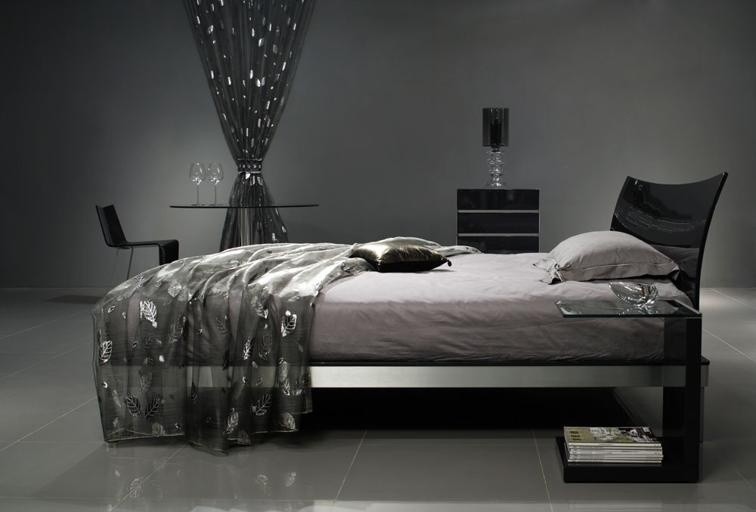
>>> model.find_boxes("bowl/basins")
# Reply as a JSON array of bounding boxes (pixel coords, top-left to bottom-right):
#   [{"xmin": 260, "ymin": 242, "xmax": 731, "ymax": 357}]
[
  {"xmin": 607, "ymin": 280, "xmax": 658, "ymax": 305},
  {"xmin": 618, "ymin": 306, "xmax": 660, "ymax": 314}
]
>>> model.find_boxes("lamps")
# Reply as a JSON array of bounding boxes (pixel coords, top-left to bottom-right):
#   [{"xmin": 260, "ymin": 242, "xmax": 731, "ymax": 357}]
[{"xmin": 482, "ymin": 109, "xmax": 509, "ymax": 191}]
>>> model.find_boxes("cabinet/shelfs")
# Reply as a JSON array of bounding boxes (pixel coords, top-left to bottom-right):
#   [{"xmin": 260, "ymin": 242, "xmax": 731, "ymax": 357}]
[{"xmin": 457, "ymin": 188, "xmax": 539, "ymax": 254}]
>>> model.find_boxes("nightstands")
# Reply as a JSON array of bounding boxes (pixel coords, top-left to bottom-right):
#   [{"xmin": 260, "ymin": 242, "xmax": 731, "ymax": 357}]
[{"xmin": 554, "ymin": 296, "xmax": 701, "ymax": 484}]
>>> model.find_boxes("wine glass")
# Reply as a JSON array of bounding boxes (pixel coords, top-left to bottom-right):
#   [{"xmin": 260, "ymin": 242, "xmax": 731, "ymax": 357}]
[
  {"xmin": 206, "ymin": 161, "xmax": 224, "ymax": 205},
  {"xmin": 188, "ymin": 160, "xmax": 205, "ymax": 206}
]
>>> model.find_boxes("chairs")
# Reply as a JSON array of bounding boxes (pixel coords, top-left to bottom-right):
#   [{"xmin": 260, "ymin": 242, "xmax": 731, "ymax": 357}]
[{"xmin": 95, "ymin": 204, "xmax": 179, "ymax": 280}]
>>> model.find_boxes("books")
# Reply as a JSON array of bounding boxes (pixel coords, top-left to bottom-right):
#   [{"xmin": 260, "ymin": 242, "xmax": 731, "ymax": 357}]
[{"xmin": 562, "ymin": 426, "xmax": 664, "ymax": 466}]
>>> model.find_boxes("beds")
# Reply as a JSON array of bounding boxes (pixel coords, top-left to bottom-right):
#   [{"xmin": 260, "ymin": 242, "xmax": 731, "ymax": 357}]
[{"xmin": 93, "ymin": 171, "xmax": 729, "ymax": 453}]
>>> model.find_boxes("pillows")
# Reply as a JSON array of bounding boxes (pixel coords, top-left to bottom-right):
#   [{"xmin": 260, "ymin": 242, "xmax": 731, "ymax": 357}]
[
  {"xmin": 535, "ymin": 231, "xmax": 679, "ymax": 286},
  {"xmin": 350, "ymin": 242, "xmax": 451, "ymax": 273}
]
